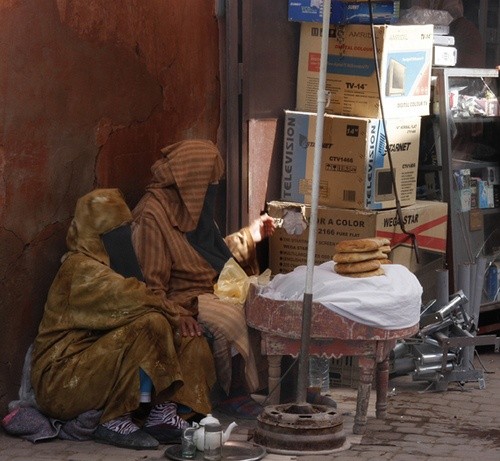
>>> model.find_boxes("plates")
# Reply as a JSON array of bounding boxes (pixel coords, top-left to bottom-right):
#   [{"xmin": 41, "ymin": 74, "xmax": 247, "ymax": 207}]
[{"xmin": 164, "ymin": 440, "xmax": 266, "ymax": 461}]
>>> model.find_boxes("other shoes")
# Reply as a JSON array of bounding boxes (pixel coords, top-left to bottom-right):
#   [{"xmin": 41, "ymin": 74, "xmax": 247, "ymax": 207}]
[
  {"xmin": 210, "ymin": 384, "xmax": 264, "ymax": 419},
  {"xmin": 143, "ymin": 423, "xmax": 191, "ymax": 444},
  {"xmin": 288, "ymin": 392, "xmax": 337, "ymax": 408},
  {"xmin": 94, "ymin": 424, "xmax": 160, "ymax": 449}
]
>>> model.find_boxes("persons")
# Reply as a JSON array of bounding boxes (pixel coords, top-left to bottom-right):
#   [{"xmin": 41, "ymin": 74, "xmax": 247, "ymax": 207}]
[
  {"xmin": 130, "ymin": 138, "xmax": 337, "ymax": 420},
  {"xmin": 22, "ymin": 184, "xmax": 206, "ymax": 449}
]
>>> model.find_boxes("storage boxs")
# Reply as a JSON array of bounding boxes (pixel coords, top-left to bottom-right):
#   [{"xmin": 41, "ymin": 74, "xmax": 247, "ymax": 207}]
[{"xmin": 266, "ymin": 0, "xmax": 500, "ymax": 275}]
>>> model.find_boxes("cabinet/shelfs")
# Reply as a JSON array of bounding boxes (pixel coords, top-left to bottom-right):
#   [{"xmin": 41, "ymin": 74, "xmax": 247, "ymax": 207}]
[{"xmin": 420, "ymin": 67, "xmax": 500, "ymax": 334}]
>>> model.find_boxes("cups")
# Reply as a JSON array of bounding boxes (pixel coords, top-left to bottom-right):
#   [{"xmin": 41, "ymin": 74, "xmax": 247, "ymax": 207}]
[
  {"xmin": 181, "ymin": 423, "xmax": 198, "ymax": 459},
  {"xmin": 203, "ymin": 423, "xmax": 222, "ymax": 461}
]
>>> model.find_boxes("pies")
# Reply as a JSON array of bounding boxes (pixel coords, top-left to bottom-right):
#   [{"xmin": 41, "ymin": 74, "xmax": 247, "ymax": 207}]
[{"xmin": 333, "ymin": 237, "xmax": 392, "ymax": 277}]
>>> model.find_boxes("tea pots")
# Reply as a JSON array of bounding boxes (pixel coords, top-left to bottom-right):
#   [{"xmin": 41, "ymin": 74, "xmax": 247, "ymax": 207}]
[{"xmin": 183, "ymin": 414, "xmax": 238, "ymax": 452}]
